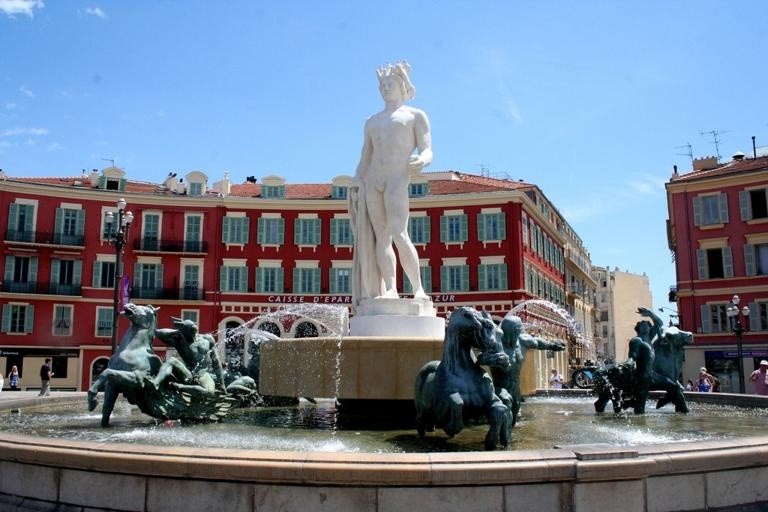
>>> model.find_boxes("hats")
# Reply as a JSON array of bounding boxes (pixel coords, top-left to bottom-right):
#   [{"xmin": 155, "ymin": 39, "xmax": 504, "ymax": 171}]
[{"xmin": 759, "ymin": 360, "xmax": 768, "ymax": 366}]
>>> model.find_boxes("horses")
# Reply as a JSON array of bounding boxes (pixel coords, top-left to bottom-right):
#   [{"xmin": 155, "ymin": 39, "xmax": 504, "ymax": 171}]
[{"xmin": 87, "ymin": 303, "xmax": 175, "ymax": 429}]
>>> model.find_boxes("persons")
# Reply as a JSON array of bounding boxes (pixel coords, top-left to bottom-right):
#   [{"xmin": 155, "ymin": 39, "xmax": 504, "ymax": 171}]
[
  {"xmin": 355, "ymin": 60, "xmax": 434, "ymax": 302},
  {"xmin": 628, "ymin": 306, "xmax": 691, "ymax": 416},
  {"xmin": 686, "ymin": 366, "xmax": 721, "ymax": 393},
  {"xmin": 549, "ymin": 367, "xmax": 565, "ymax": 389},
  {"xmin": 479, "ymin": 315, "xmax": 565, "ymax": 429},
  {"xmin": 8, "ymin": 365, "xmax": 20, "ymax": 391},
  {"xmin": 37, "ymin": 358, "xmax": 55, "ymax": 397},
  {"xmin": 748, "ymin": 359, "xmax": 768, "ymax": 395}
]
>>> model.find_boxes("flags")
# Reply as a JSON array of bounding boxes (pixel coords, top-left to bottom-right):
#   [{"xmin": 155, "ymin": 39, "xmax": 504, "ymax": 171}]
[{"xmin": 118, "ymin": 272, "xmax": 133, "ymax": 316}]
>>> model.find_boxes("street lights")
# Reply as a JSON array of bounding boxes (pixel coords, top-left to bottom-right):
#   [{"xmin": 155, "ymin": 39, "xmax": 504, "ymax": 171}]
[
  {"xmin": 581, "ymin": 276, "xmax": 590, "ymax": 361},
  {"xmin": 590, "ymin": 285, "xmax": 602, "ymax": 359},
  {"xmin": 725, "ymin": 295, "xmax": 751, "ymax": 392},
  {"xmin": 104, "ymin": 198, "xmax": 134, "ymax": 356}
]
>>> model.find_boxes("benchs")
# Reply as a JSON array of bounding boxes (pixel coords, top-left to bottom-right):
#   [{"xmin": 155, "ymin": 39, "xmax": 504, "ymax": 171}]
[{"xmin": 21, "ymin": 386, "xmax": 77, "ymax": 392}]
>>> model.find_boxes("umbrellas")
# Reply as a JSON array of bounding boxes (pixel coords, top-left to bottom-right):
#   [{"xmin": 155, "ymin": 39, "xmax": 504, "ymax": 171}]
[{"xmin": 582, "ymin": 370, "xmax": 593, "ymax": 379}]
